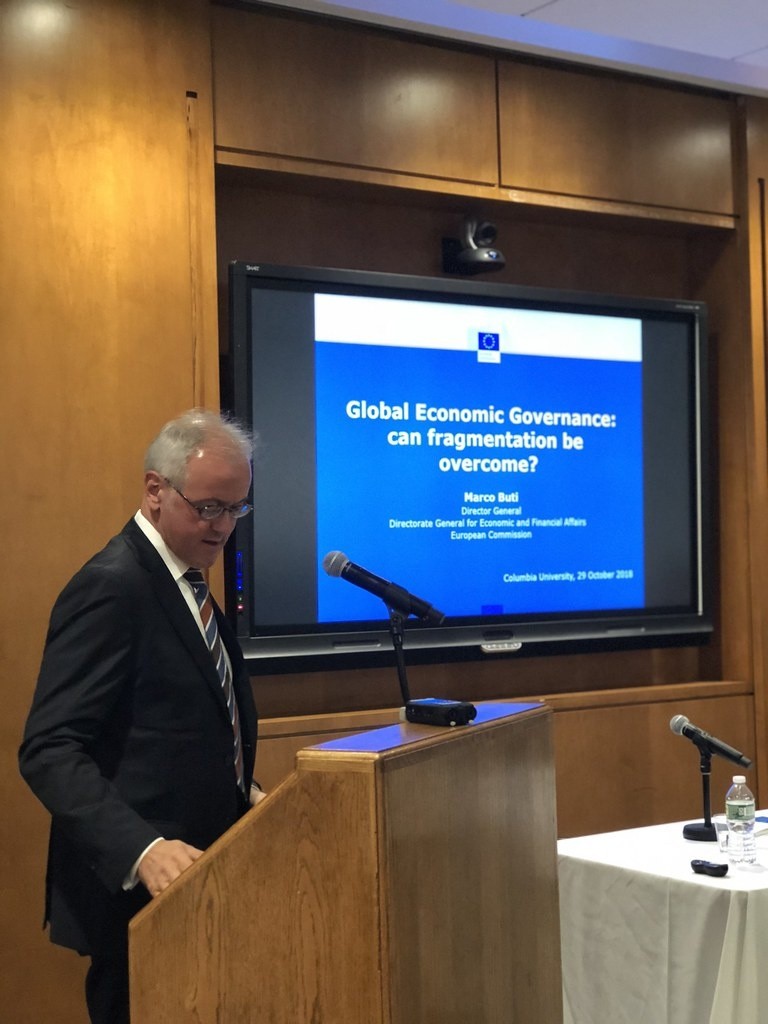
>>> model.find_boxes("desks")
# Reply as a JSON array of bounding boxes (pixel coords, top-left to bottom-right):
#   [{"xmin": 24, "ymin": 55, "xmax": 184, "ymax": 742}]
[{"xmin": 555, "ymin": 809, "xmax": 768, "ymax": 1024}]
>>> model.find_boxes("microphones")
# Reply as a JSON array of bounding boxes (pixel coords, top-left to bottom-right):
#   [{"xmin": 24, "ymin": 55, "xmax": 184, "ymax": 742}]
[
  {"xmin": 322, "ymin": 551, "xmax": 446, "ymax": 626},
  {"xmin": 669, "ymin": 714, "xmax": 752, "ymax": 768}
]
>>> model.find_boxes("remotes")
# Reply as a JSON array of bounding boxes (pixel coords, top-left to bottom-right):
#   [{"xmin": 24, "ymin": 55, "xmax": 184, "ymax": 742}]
[{"xmin": 690, "ymin": 860, "xmax": 728, "ymax": 878}]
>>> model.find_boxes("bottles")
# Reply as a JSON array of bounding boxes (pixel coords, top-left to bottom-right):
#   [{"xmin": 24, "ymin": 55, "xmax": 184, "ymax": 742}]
[{"xmin": 725, "ymin": 775, "xmax": 757, "ymax": 864}]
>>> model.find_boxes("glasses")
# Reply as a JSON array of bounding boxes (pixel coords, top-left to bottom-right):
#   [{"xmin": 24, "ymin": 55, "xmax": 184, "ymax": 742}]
[{"xmin": 164, "ymin": 478, "xmax": 253, "ymax": 521}]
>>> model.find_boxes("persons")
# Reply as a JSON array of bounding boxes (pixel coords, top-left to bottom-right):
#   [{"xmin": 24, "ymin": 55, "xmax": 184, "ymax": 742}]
[{"xmin": 18, "ymin": 411, "xmax": 267, "ymax": 1024}]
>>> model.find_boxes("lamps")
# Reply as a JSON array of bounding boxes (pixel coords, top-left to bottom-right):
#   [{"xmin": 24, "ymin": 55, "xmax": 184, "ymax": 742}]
[{"xmin": 440, "ymin": 214, "xmax": 506, "ymax": 273}]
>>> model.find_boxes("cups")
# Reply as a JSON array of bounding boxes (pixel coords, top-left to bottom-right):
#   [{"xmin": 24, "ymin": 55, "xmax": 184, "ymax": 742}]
[{"xmin": 713, "ymin": 813, "xmax": 729, "ymax": 852}]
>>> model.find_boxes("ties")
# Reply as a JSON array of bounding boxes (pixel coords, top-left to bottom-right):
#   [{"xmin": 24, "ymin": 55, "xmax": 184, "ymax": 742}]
[{"xmin": 182, "ymin": 566, "xmax": 245, "ymax": 803}]
